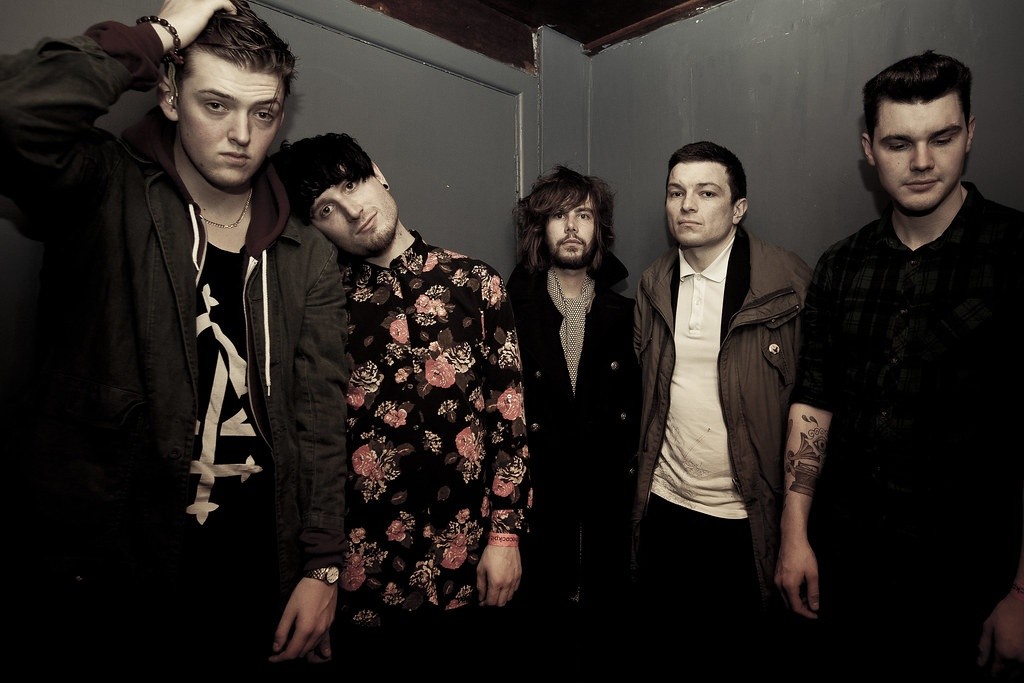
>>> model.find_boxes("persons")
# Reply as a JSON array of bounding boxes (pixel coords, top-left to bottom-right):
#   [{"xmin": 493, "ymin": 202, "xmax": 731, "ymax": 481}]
[
  {"xmin": 506, "ymin": 164, "xmax": 643, "ymax": 619},
  {"xmin": 269, "ymin": 132, "xmax": 534, "ymax": 664},
  {"xmin": 774, "ymin": 50, "xmax": 1024, "ymax": 683},
  {"xmin": 633, "ymin": 142, "xmax": 814, "ymax": 593},
  {"xmin": 0, "ymin": 0, "xmax": 351, "ymax": 683}
]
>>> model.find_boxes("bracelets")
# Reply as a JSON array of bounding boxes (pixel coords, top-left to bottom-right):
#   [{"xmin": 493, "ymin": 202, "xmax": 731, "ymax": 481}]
[
  {"xmin": 1011, "ymin": 581, "xmax": 1024, "ymax": 602},
  {"xmin": 135, "ymin": 16, "xmax": 185, "ymax": 64},
  {"xmin": 489, "ymin": 532, "xmax": 519, "ymax": 547}
]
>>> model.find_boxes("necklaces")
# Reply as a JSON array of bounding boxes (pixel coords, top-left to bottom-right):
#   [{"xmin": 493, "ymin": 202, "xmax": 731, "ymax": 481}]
[{"xmin": 199, "ymin": 180, "xmax": 253, "ymax": 229}]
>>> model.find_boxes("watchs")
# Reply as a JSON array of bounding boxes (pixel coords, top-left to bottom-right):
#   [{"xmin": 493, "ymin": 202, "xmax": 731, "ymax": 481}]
[{"xmin": 304, "ymin": 566, "xmax": 340, "ymax": 586}]
